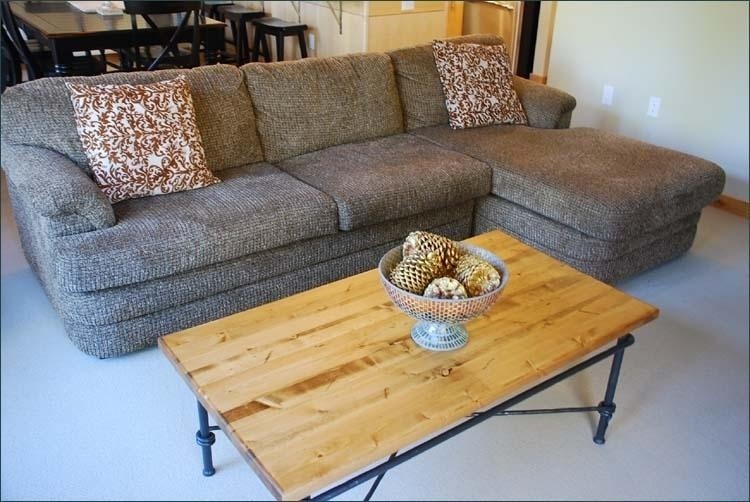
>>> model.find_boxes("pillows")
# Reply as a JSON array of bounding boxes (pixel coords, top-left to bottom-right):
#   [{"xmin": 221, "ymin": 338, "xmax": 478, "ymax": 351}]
[
  {"xmin": 429, "ymin": 38, "xmax": 529, "ymax": 131},
  {"xmin": 62, "ymin": 74, "xmax": 224, "ymax": 204}
]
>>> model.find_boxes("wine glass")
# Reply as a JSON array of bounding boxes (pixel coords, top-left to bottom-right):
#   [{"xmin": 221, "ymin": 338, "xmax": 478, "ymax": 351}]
[{"xmin": 377, "ymin": 240, "xmax": 511, "ymax": 354}]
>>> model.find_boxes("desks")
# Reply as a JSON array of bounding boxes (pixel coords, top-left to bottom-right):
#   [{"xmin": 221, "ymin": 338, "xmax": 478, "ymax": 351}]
[{"xmin": 157, "ymin": 228, "xmax": 662, "ymax": 502}]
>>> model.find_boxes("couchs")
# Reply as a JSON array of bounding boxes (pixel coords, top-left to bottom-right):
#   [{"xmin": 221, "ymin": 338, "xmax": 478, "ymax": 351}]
[{"xmin": 0, "ymin": 34, "xmax": 727, "ymax": 357}]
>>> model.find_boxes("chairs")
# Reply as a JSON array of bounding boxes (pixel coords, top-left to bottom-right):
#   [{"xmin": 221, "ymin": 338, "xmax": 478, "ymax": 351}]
[{"xmin": 0, "ymin": 0, "xmax": 310, "ymax": 89}]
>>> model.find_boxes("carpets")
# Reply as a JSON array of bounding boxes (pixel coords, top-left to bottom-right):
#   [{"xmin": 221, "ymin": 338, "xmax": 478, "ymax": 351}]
[{"xmin": 0, "ymin": 205, "xmax": 749, "ymax": 502}]
